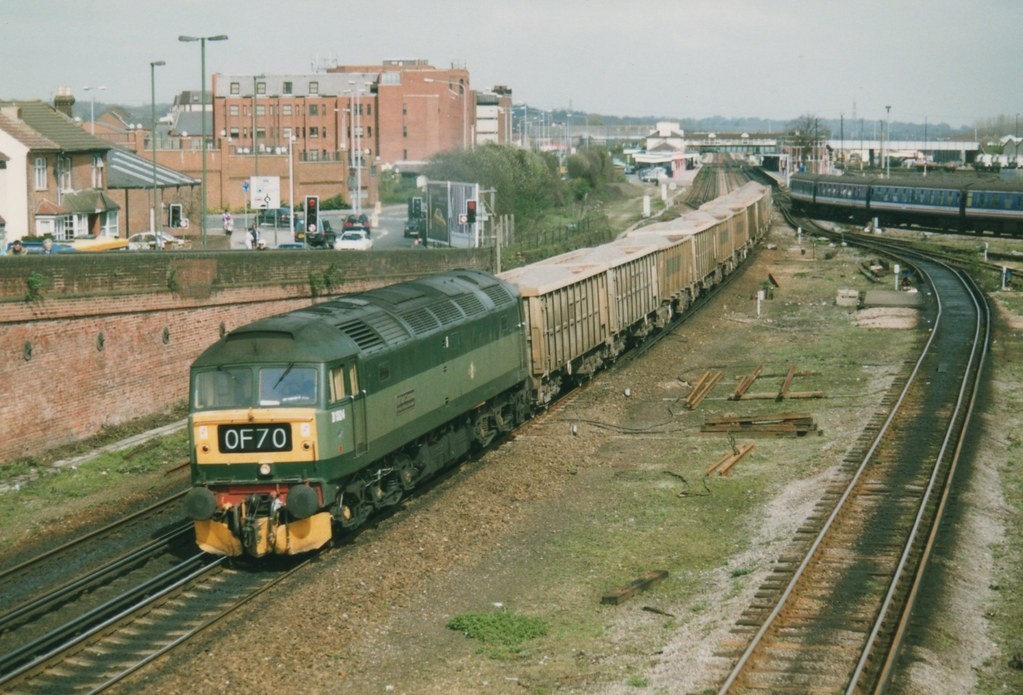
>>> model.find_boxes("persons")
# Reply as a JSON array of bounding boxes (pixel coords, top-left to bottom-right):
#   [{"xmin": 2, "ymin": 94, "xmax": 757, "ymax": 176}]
[
  {"xmin": 221, "ymin": 209, "xmax": 232, "ymax": 237},
  {"xmin": 37, "ymin": 238, "xmax": 57, "ymax": 256},
  {"xmin": 250, "ymin": 224, "xmax": 260, "ymax": 246},
  {"xmin": 245, "ymin": 228, "xmax": 254, "ymax": 248},
  {"xmin": 6, "ymin": 240, "xmax": 29, "ymax": 255}
]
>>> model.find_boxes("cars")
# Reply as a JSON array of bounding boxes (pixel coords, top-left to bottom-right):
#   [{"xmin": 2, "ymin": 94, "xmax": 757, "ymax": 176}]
[
  {"xmin": 294, "ymin": 217, "xmax": 336, "ymax": 249},
  {"xmin": 332, "ymin": 229, "xmax": 373, "ymax": 251},
  {"xmin": 274, "ymin": 242, "xmax": 307, "ymax": 250},
  {"xmin": 120, "ymin": 232, "xmax": 191, "ymax": 252},
  {"xmin": 255, "ymin": 208, "xmax": 300, "ymax": 227},
  {"xmin": 623, "ymin": 164, "xmax": 657, "ymax": 182},
  {"xmin": 341, "ymin": 212, "xmax": 371, "ymax": 234},
  {"xmin": 403, "ymin": 216, "xmax": 427, "ymax": 237}
]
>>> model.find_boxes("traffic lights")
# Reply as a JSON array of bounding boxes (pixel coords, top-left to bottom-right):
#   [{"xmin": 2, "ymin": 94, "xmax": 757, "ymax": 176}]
[
  {"xmin": 414, "ymin": 196, "xmax": 421, "ymax": 216},
  {"xmin": 306, "ymin": 196, "xmax": 318, "ymax": 232},
  {"xmin": 466, "ymin": 199, "xmax": 476, "ymax": 221}
]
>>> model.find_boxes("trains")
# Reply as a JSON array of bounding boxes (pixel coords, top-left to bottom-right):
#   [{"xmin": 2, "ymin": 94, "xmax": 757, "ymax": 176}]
[
  {"xmin": 185, "ymin": 178, "xmax": 775, "ymax": 567},
  {"xmin": 790, "ymin": 168, "xmax": 1023, "ymax": 229}
]
